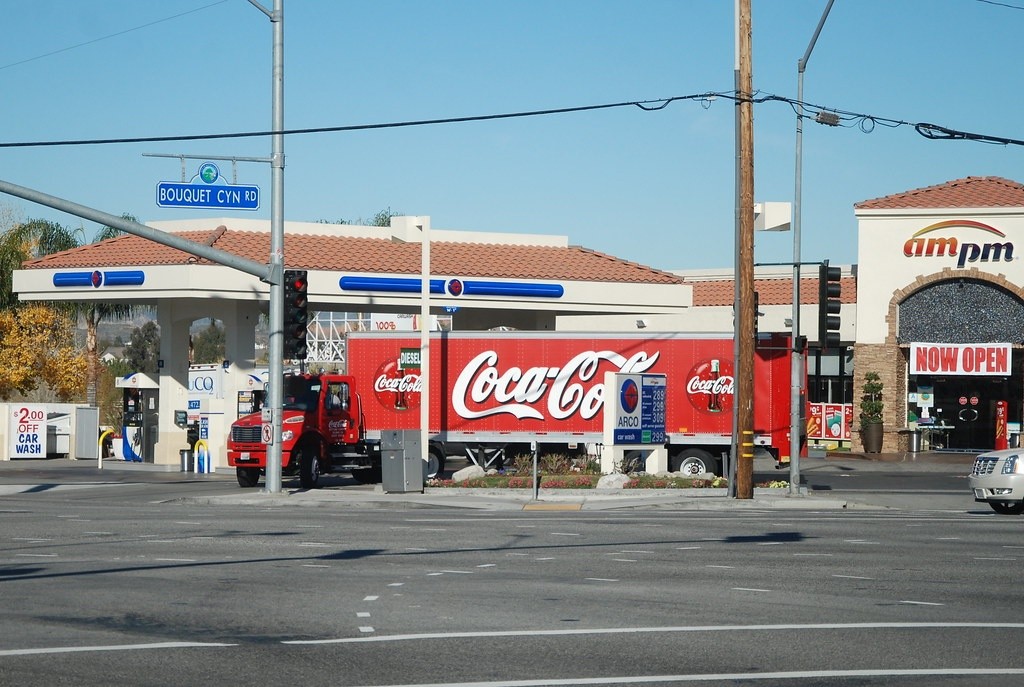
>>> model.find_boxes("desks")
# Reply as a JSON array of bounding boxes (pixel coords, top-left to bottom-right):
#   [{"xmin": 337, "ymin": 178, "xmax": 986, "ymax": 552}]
[{"xmin": 917, "ymin": 426, "xmax": 956, "ymax": 449}]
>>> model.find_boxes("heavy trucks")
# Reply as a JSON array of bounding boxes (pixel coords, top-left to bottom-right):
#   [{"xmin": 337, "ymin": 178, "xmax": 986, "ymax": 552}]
[{"xmin": 224, "ymin": 330, "xmax": 813, "ymax": 491}]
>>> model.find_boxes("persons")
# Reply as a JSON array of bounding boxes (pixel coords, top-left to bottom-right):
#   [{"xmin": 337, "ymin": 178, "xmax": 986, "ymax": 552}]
[{"xmin": 325, "ymin": 386, "xmax": 341, "ymax": 409}]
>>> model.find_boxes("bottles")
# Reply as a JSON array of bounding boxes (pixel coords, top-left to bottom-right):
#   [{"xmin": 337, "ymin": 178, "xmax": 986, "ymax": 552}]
[
  {"xmin": 707, "ymin": 360, "xmax": 723, "ymax": 412},
  {"xmin": 394, "ymin": 358, "xmax": 409, "ymax": 410}
]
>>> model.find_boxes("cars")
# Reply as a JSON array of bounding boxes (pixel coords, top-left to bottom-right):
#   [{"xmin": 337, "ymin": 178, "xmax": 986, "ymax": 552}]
[{"xmin": 969, "ymin": 446, "xmax": 1024, "ymax": 515}]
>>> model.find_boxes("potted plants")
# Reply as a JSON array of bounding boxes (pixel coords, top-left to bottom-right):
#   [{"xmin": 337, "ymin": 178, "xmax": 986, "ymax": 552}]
[{"xmin": 860, "ymin": 372, "xmax": 884, "ymax": 454}]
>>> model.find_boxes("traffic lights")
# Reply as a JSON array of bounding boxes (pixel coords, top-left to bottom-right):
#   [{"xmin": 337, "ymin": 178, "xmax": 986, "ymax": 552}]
[
  {"xmin": 284, "ymin": 269, "xmax": 308, "ymax": 360},
  {"xmin": 817, "ymin": 266, "xmax": 841, "ymax": 349}
]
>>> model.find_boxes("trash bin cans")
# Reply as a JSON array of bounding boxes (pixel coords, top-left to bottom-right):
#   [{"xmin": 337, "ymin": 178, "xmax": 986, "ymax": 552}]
[
  {"xmin": 909, "ymin": 431, "xmax": 922, "ymax": 453},
  {"xmin": 180, "ymin": 449, "xmax": 193, "ymax": 471}
]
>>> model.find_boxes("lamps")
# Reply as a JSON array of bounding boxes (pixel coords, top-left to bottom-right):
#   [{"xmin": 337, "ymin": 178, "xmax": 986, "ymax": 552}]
[
  {"xmin": 223, "ymin": 359, "xmax": 229, "ymax": 368},
  {"xmin": 636, "ymin": 319, "xmax": 648, "ymax": 328},
  {"xmin": 158, "ymin": 359, "xmax": 164, "ymax": 368},
  {"xmin": 785, "ymin": 317, "xmax": 792, "ymax": 326}
]
